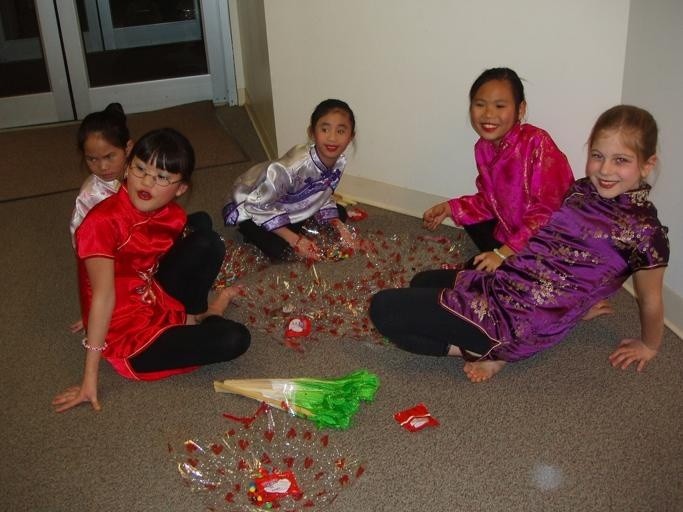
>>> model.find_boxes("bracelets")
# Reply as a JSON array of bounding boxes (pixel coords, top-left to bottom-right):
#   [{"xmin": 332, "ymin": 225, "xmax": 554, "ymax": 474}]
[
  {"xmin": 81, "ymin": 338, "xmax": 108, "ymax": 351},
  {"xmin": 493, "ymin": 249, "xmax": 508, "ymax": 261},
  {"xmin": 294, "ymin": 237, "xmax": 303, "ymax": 251}
]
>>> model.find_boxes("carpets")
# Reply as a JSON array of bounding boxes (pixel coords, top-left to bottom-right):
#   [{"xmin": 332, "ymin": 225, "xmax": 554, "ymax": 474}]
[{"xmin": 0, "ymin": 100, "xmax": 251, "ymax": 204}]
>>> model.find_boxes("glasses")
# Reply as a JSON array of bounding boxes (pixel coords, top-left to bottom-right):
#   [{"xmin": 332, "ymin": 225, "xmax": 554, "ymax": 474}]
[{"xmin": 128, "ymin": 161, "xmax": 185, "ymax": 187}]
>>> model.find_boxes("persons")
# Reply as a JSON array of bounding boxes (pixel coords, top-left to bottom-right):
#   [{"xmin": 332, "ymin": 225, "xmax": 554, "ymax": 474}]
[
  {"xmin": 70, "ymin": 103, "xmax": 212, "ymax": 336},
  {"xmin": 370, "ymin": 104, "xmax": 670, "ymax": 384},
  {"xmin": 53, "ymin": 129, "xmax": 252, "ymax": 414},
  {"xmin": 420, "ymin": 67, "xmax": 576, "ymax": 272},
  {"xmin": 222, "ymin": 99, "xmax": 355, "ymax": 264}
]
